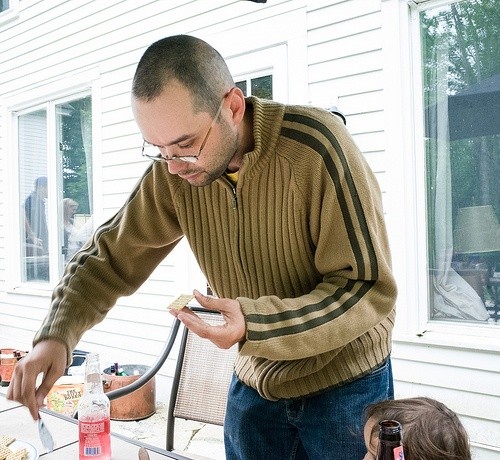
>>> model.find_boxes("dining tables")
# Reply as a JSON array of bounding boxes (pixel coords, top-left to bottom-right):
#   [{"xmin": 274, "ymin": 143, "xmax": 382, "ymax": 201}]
[{"xmin": 0, "ymin": 393, "xmax": 193, "ymax": 460}]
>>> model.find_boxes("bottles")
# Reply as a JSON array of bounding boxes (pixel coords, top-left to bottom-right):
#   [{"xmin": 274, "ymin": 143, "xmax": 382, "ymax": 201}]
[
  {"xmin": 118, "ymin": 368, "xmax": 129, "ymax": 376},
  {"xmin": 111, "ymin": 365, "xmax": 116, "ymax": 375},
  {"xmin": 376, "ymin": 420, "xmax": 405, "ymax": 460},
  {"xmin": 13, "ymin": 351, "xmax": 20, "ymax": 358},
  {"xmin": 78, "ymin": 352, "xmax": 111, "ymax": 460}
]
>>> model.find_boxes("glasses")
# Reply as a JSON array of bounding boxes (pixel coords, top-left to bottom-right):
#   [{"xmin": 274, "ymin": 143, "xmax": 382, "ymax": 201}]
[{"xmin": 141, "ymin": 93, "xmax": 228, "ymax": 163}]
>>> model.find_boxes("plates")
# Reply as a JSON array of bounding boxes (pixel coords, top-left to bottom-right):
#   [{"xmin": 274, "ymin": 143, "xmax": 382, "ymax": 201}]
[{"xmin": 7, "ymin": 439, "xmax": 39, "ymax": 460}]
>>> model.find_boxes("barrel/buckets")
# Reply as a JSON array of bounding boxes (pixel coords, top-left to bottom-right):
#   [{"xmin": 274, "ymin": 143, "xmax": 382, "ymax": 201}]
[
  {"xmin": 0, "ymin": 349, "xmax": 26, "ymax": 382},
  {"xmin": 103, "ymin": 364, "xmax": 155, "ymax": 420}
]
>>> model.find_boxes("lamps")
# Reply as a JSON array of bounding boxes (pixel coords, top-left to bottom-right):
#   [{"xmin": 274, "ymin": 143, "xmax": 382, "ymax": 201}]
[{"xmin": 453, "ymin": 204, "xmax": 500, "ymax": 287}]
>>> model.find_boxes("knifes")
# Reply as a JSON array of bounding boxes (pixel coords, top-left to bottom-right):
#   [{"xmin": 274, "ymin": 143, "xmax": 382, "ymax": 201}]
[{"xmin": 36, "ymin": 412, "xmax": 54, "ymax": 450}]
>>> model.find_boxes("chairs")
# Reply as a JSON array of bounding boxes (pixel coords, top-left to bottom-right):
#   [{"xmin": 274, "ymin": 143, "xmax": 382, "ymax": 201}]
[{"xmin": 73, "ymin": 307, "xmax": 241, "ymax": 460}]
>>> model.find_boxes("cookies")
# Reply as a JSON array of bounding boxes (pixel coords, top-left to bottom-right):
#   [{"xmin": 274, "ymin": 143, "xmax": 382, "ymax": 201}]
[
  {"xmin": 0, "ymin": 434, "xmax": 27, "ymax": 460},
  {"xmin": 166, "ymin": 295, "xmax": 195, "ymax": 310}
]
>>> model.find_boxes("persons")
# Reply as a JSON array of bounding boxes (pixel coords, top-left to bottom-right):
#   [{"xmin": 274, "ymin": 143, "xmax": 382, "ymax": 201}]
[
  {"xmin": 25, "ymin": 177, "xmax": 50, "ymax": 282},
  {"xmin": 63, "ymin": 198, "xmax": 78, "ymax": 260},
  {"xmin": 362, "ymin": 396, "xmax": 472, "ymax": 460},
  {"xmin": 7, "ymin": 33, "xmax": 399, "ymax": 460}
]
